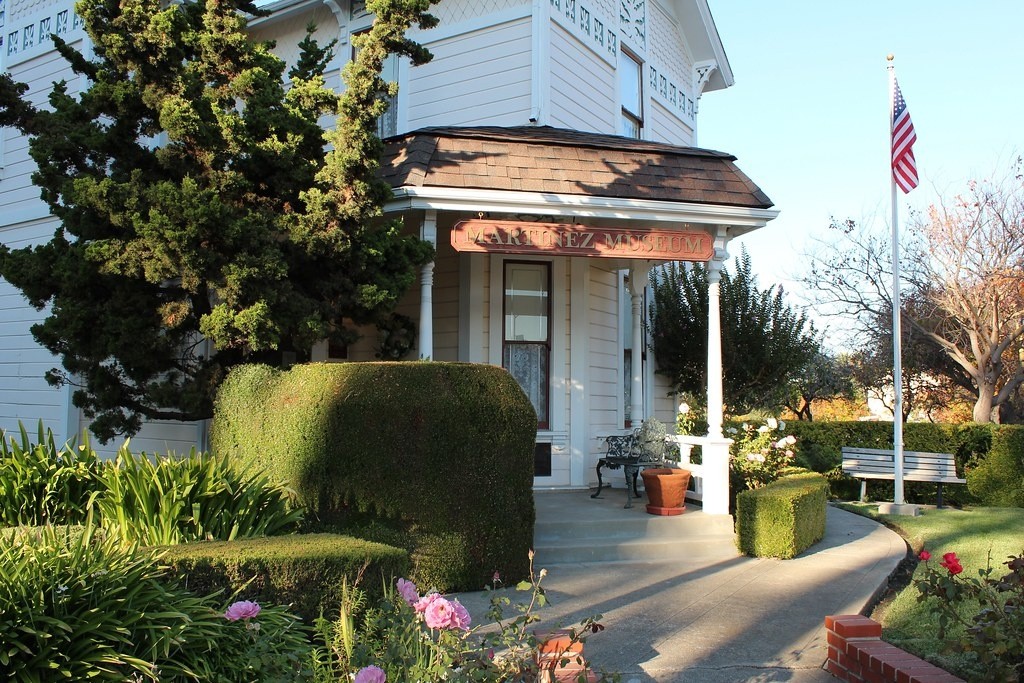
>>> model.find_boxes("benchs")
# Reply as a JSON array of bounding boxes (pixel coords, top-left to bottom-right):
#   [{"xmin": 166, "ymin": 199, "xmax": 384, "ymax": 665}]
[{"xmin": 842, "ymin": 447, "xmax": 966, "ymax": 508}]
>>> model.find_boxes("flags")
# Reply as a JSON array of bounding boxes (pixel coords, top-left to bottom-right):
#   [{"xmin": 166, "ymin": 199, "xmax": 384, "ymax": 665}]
[{"xmin": 891, "ymin": 74, "xmax": 919, "ymax": 194}]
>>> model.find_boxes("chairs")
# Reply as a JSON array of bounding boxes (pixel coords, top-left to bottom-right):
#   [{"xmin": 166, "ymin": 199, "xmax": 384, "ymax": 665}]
[{"xmin": 591, "ymin": 417, "xmax": 666, "ymax": 509}]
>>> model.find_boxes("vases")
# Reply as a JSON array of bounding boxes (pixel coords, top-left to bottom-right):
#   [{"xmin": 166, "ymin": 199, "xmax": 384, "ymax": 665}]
[{"xmin": 640, "ymin": 468, "xmax": 691, "ymax": 516}]
